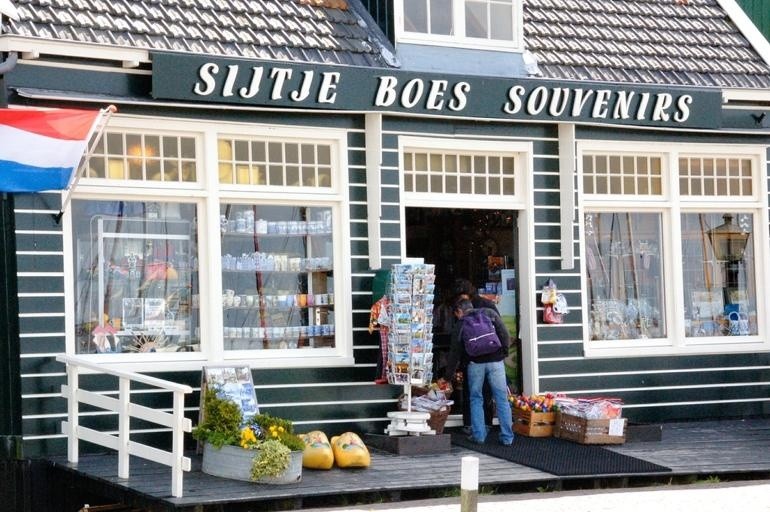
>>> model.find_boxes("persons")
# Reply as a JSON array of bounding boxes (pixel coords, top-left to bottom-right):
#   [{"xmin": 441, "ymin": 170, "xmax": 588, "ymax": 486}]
[
  {"xmin": 452, "ymin": 277, "xmax": 500, "ymax": 436},
  {"xmin": 436, "ymin": 291, "xmax": 514, "ymax": 447}
]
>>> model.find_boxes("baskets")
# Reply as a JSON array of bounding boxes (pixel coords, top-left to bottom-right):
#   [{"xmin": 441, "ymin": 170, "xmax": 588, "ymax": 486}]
[{"xmin": 399, "ymin": 404, "xmax": 451, "ymax": 434}]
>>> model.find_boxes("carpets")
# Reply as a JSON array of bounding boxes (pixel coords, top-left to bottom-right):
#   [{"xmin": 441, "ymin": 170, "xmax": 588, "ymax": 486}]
[{"xmin": 447, "ymin": 421, "xmax": 674, "ymax": 476}]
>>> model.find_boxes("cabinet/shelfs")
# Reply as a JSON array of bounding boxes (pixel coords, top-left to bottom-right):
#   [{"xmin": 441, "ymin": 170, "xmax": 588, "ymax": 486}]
[
  {"xmin": 681, "ymin": 226, "xmax": 716, "ymax": 330},
  {"xmin": 585, "ymin": 228, "xmax": 619, "ymax": 311},
  {"xmin": 216, "ymin": 226, "xmax": 335, "ymax": 353},
  {"xmin": 614, "ymin": 226, "xmax": 661, "ymax": 312},
  {"xmin": 383, "ymin": 257, "xmax": 438, "ymax": 439}
]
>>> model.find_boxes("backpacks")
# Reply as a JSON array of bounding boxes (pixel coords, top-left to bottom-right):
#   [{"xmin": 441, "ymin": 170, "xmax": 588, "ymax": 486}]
[{"xmin": 460, "ymin": 307, "xmax": 501, "ymax": 356}]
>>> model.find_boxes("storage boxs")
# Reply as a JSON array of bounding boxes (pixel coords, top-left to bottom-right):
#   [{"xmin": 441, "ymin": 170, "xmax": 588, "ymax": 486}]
[
  {"xmin": 512, "ymin": 408, "xmax": 556, "ymax": 441},
  {"xmin": 553, "ymin": 411, "xmax": 629, "ymax": 448}
]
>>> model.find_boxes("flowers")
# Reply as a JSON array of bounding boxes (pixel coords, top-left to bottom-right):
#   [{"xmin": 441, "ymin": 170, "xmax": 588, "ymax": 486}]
[{"xmin": 235, "ymin": 418, "xmax": 283, "ymax": 451}]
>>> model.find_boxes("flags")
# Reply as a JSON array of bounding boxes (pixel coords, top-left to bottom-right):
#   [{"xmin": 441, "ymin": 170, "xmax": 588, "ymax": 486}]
[{"xmin": 0, "ymin": 107, "xmax": 105, "ymax": 195}]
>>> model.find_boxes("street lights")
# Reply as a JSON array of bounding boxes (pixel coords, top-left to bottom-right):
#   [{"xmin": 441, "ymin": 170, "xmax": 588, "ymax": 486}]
[{"xmin": 705, "ymin": 213, "xmax": 752, "ymax": 337}]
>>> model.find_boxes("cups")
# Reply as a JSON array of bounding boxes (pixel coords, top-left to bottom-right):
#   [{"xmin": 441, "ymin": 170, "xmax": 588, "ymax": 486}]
[
  {"xmin": 221, "ymin": 209, "xmax": 331, "ymax": 236},
  {"xmin": 223, "ymin": 323, "xmax": 334, "ymax": 340},
  {"xmin": 221, "ymin": 251, "xmax": 330, "ymax": 271},
  {"xmin": 223, "ymin": 289, "xmax": 333, "ymax": 309}
]
